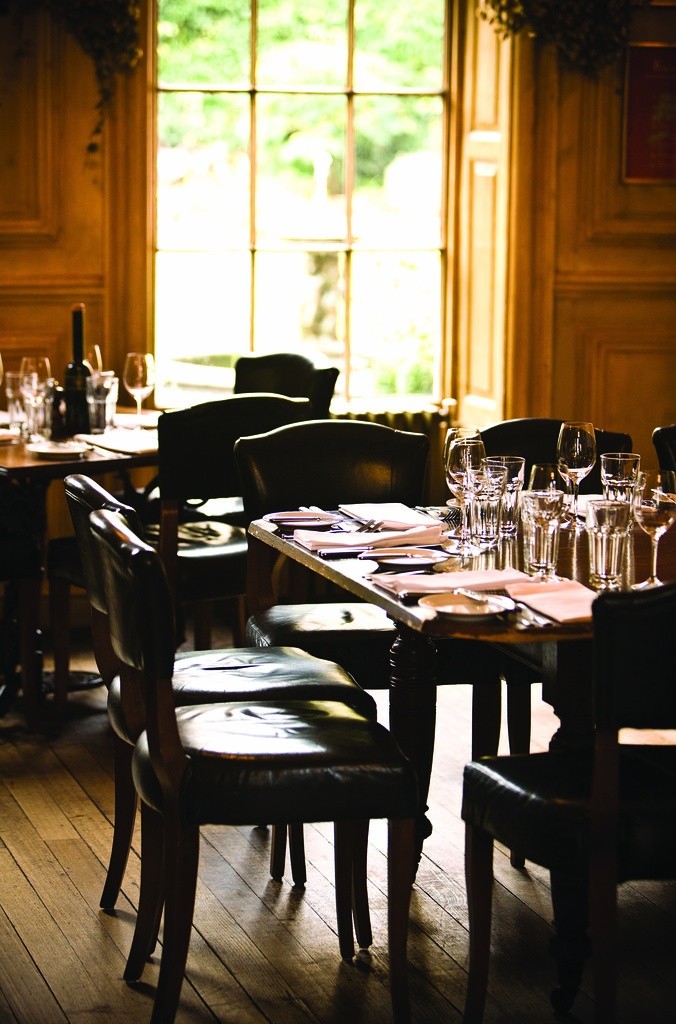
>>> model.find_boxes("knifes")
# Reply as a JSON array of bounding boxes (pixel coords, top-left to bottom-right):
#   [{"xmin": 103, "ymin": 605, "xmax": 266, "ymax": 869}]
[
  {"xmin": 457, "ymin": 588, "xmax": 514, "ymax": 610},
  {"xmin": 358, "ymin": 554, "xmax": 458, "ymax": 560},
  {"xmin": 269, "ymin": 517, "xmax": 369, "ymax": 522}
]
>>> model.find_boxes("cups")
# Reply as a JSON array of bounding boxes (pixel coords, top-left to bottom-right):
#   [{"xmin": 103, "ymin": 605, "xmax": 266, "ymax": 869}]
[
  {"xmin": 600, "ymin": 453, "xmax": 640, "ymax": 530},
  {"xmin": 521, "ymin": 491, "xmax": 564, "ymax": 575},
  {"xmin": 482, "ymin": 457, "xmax": 525, "ymax": 536},
  {"xmin": 586, "ymin": 500, "xmax": 630, "ymax": 588},
  {"xmin": 85, "ymin": 377, "xmax": 119, "ymax": 434},
  {"xmin": 6, "ymin": 372, "xmax": 37, "ymax": 439},
  {"xmin": 467, "ymin": 466, "xmax": 507, "ymax": 542}
]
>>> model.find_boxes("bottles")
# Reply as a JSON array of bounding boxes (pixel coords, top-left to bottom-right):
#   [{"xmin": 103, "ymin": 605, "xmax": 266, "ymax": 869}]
[{"xmin": 52, "ymin": 305, "xmax": 92, "ymax": 441}]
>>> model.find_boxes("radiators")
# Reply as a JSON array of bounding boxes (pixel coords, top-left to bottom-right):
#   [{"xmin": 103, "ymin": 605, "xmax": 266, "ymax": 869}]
[{"xmin": 328, "ymin": 397, "xmax": 454, "ymax": 500}]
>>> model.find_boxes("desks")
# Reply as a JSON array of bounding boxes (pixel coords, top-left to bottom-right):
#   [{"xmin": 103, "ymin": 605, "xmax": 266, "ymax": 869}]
[
  {"xmin": 248, "ymin": 508, "xmax": 675, "ymax": 882},
  {"xmin": 0, "ymin": 411, "xmax": 159, "ymax": 714}
]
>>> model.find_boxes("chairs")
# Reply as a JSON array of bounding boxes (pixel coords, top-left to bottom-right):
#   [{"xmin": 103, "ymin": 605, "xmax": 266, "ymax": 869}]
[{"xmin": 0, "ymin": 352, "xmax": 676, "ymax": 1024}]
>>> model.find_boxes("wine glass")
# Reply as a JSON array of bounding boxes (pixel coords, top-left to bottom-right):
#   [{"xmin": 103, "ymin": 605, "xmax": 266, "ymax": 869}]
[
  {"xmin": 124, "ymin": 353, "xmax": 156, "ymax": 430},
  {"xmin": 631, "ymin": 471, "xmax": 676, "ymax": 592},
  {"xmin": 556, "ymin": 422, "xmax": 596, "ymax": 531},
  {"xmin": 529, "ymin": 465, "xmax": 571, "ymax": 574},
  {"xmin": 20, "ymin": 357, "xmax": 51, "ymax": 444},
  {"xmin": 444, "ymin": 428, "xmax": 488, "ymax": 555}
]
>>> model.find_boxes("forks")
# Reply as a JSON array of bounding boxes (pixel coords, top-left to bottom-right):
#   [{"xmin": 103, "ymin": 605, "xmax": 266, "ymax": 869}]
[
  {"xmin": 415, "ymin": 506, "xmax": 459, "ymax": 521},
  {"xmin": 282, "ymin": 519, "xmax": 384, "ymax": 539},
  {"xmin": 485, "ymin": 589, "xmax": 554, "ymax": 628},
  {"xmin": 299, "ymin": 506, "xmax": 384, "ymax": 533}
]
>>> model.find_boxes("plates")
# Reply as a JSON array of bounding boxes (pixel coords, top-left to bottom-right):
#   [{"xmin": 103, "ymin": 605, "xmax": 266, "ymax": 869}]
[
  {"xmin": 262, "ymin": 511, "xmax": 343, "ymax": 527},
  {"xmin": 366, "ymin": 548, "xmax": 450, "ymax": 570},
  {"xmin": 445, "ymin": 497, "xmax": 460, "ymax": 508},
  {"xmin": 28, "ymin": 443, "xmax": 87, "ymax": 455},
  {"xmin": 418, "ymin": 593, "xmax": 516, "ymax": 622}
]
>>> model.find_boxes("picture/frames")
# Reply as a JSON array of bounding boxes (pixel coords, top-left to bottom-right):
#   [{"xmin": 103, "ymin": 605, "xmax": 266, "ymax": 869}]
[{"xmin": 620, "ymin": 41, "xmax": 675, "ymax": 186}]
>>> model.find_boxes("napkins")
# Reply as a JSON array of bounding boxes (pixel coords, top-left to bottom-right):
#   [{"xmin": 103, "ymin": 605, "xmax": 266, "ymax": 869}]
[
  {"xmin": 505, "ymin": 581, "xmax": 598, "ymax": 623},
  {"xmin": 107, "ymin": 412, "xmax": 162, "ymax": 429},
  {"xmin": 338, "ymin": 503, "xmax": 446, "ymax": 530},
  {"xmin": 561, "ymin": 493, "xmax": 603, "ymax": 518},
  {"xmin": 73, "ymin": 426, "xmax": 160, "ymax": 454},
  {"xmin": 293, "ymin": 526, "xmax": 450, "ymax": 551},
  {"xmin": 372, "ymin": 567, "xmax": 529, "ymax": 598}
]
